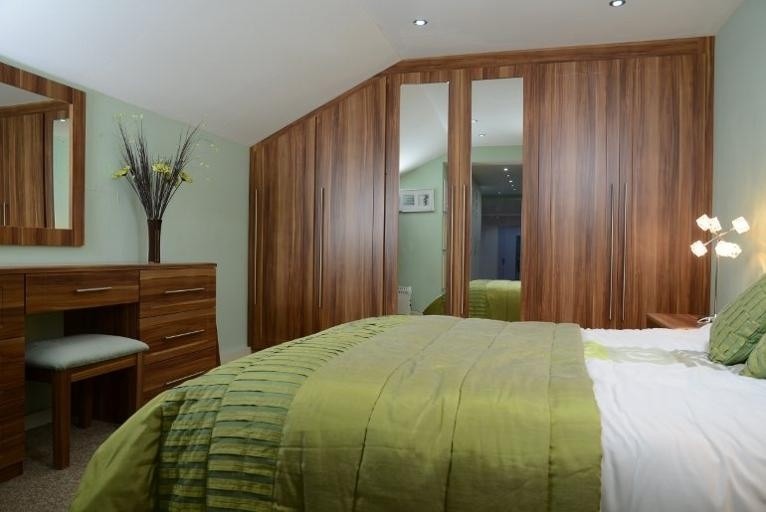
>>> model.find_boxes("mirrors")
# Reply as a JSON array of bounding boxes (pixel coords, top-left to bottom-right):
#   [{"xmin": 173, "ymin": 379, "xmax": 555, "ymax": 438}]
[
  {"xmin": 397, "ymin": 81, "xmax": 450, "ymax": 318},
  {"xmin": 0, "ymin": 62, "xmax": 87, "ymax": 248},
  {"xmin": 464, "ymin": 77, "xmax": 524, "ymax": 322}
]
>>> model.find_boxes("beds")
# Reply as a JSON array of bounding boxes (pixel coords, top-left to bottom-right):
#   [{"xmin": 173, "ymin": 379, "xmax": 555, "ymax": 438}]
[
  {"xmin": 422, "ymin": 273, "xmax": 524, "ymax": 320},
  {"xmin": 68, "ymin": 313, "xmax": 765, "ymax": 511}
]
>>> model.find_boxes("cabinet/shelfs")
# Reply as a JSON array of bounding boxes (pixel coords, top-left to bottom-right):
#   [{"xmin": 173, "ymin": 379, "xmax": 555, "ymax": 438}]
[
  {"xmin": 246, "ymin": 76, "xmax": 387, "ymax": 349},
  {"xmin": 0, "ymin": 104, "xmax": 53, "ymax": 227},
  {"xmin": 530, "ymin": 45, "xmax": 705, "ymax": 330}
]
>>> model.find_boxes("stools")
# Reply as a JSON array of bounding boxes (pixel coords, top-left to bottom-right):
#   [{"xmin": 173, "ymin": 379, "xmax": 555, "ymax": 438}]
[{"xmin": 23, "ymin": 333, "xmax": 152, "ymax": 471}]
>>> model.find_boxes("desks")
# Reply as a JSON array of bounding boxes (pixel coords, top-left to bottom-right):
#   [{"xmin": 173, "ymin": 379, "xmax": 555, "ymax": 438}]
[{"xmin": 0, "ymin": 262, "xmax": 220, "ymax": 481}]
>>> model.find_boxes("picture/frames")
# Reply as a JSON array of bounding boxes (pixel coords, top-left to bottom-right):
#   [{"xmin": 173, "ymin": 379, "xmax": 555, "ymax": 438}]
[{"xmin": 397, "ymin": 188, "xmax": 436, "ymax": 215}]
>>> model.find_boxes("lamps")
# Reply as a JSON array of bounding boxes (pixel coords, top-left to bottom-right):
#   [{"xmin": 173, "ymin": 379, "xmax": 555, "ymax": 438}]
[{"xmin": 690, "ymin": 210, "xmax": 751, "ymax": 322}]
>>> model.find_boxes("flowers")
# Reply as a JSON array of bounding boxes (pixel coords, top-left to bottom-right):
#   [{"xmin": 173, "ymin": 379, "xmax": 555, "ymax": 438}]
[{"xmin": 109, "ymin": 110, "xmax": 219, "ymax": 220}]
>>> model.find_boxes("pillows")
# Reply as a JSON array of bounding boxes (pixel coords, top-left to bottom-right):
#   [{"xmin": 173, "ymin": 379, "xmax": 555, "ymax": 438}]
[
  {"xmin": 737, "ymin": 330, "xmax": 765, "ymax": 380},
  {"xmin": 708, "ymin": 270, "xmax": 765, "ymax": 366}
]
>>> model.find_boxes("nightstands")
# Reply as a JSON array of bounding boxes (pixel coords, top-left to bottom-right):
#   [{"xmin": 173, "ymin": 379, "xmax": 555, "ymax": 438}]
[{"xmin": 645, "ymin": 312, "xmax": 701, "ymax": 332}]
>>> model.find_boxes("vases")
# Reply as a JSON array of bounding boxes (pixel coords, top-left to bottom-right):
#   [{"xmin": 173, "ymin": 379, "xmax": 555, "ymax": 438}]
[{"xmin": 145, "ymin": 219, "xmax": 162, "ymax": 264}]
